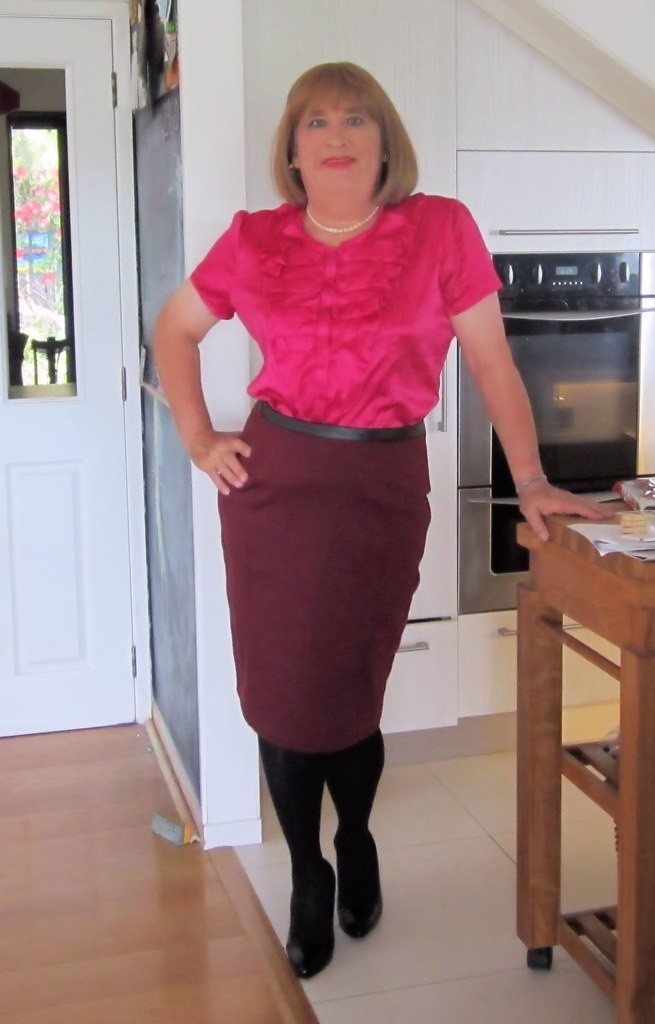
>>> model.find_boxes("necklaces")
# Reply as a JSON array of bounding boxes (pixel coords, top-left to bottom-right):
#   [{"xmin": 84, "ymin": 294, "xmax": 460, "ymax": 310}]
[{"xmin": 304, "ymin": 203, "xmax": 379, "ymax": 234}]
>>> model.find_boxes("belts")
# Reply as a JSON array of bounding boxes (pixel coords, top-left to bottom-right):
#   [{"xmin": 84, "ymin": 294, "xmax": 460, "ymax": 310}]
[{"xmin": 258, "ymin": 399, "xmax": 428, "ymax": 441}]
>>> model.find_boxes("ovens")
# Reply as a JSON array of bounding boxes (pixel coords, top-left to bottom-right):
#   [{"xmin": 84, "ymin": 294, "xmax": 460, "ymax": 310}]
[{"xmin": 456, "ymin": 251, "xmax": 642, "ymax": 616}]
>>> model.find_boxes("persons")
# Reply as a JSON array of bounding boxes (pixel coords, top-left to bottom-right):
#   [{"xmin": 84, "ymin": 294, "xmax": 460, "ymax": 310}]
[{"xmin": 150, "ymin": 61, "xmax": 617, "ymax": 981}]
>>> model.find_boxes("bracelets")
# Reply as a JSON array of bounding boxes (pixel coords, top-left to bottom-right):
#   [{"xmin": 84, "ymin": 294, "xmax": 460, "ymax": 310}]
[{"xmin": 514, "ymin": 475, "xmax": 547, "ymax": 494}]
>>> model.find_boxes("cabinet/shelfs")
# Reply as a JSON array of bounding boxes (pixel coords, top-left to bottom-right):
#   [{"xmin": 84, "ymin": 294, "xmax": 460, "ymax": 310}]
[{"xmin": 507, "ymin": 499, "xmax": 654, "ymax": 1023}]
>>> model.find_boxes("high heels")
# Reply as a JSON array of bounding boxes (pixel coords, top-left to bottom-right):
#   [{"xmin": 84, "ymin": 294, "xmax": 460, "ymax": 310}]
[
  {"xmin": 334, "ymin": 829, "xmax": 382, "ymax": 937},
  {"xmin": 287, "ymin": 858, "xmax": 335, "ymax": 976}
]
